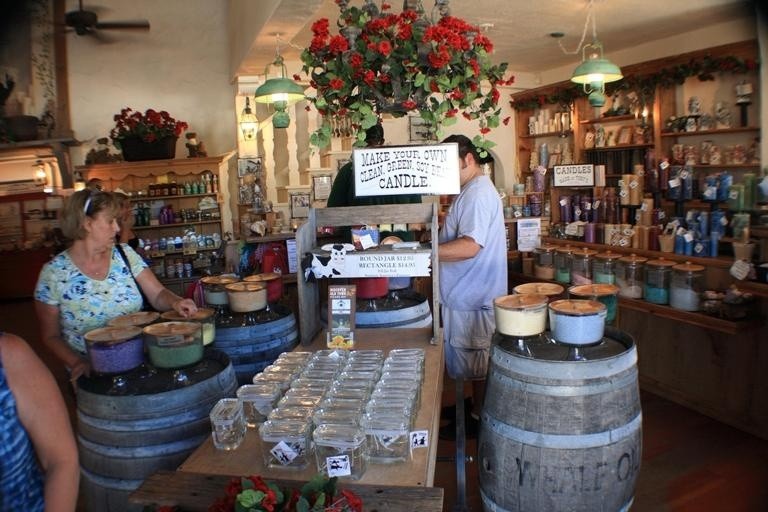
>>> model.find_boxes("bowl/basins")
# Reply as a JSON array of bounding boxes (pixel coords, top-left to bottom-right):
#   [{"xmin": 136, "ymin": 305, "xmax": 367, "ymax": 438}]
[
  {"xmin": 350, "ymin": 277, "xmax": 411, "ymax": 300},
  {"xmin": 494, "ymin": 282, "xmax": 618, "ymax": 344},
  {"xmin": 84, "ymin": 309, "xmax": 216, "ymax": 376},
  {"xmin": 202, "ymin": 274, "xmax": 282, "ymax": 312}
]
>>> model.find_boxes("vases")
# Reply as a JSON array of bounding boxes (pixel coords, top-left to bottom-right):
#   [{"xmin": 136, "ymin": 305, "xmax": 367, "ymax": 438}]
[{"xmin": 119, "ymin": 136, "xmax": 179, "ymax": 161}]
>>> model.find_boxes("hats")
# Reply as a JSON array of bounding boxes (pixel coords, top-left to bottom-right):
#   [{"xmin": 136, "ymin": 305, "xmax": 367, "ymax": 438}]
[{"xmin": 363, "ymin": 124, "xmax": 385, "ymax": 141}]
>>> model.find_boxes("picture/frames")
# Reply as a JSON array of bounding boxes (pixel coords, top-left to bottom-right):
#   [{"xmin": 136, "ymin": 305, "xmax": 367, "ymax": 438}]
[
  {"xmin": 337, "ymin": 159, "xmax": 351, "ymax": 171},
  {"xmin": 0, "ymin": 0, "xmax": 71, "ymax": 149},
  {"xmin": 313, "ymin": 175, "xmax": 332, "ymax": 201},
  {"xmin": 290, "ymin": 194, "xmax": 311, "ymax": 219},
  {"xmin": 237, "ymin": 157, "xmax": 263, "ymax": 179},
  {"xmin": 408, "ymin": 116, "xmax": 439, "ymax": 141}
]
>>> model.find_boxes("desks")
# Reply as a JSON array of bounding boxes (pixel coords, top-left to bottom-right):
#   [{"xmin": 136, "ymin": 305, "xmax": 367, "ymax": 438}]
[{"xmin": 124, "ymin": 328, "xmax": 446, "ymax": 512}]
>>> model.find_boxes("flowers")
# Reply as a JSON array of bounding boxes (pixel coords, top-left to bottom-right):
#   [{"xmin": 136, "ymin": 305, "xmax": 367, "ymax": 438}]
[
  {"xmin": 140, "ymin": 474, "xmax": 362, "ymax": 512},
  {"xmin": 110, "ymin": 106, "xmax": 189, "ymax": 142},
  {"xmin": 301, "ymin": 0, "xmax": 516, "ymax": 160}
]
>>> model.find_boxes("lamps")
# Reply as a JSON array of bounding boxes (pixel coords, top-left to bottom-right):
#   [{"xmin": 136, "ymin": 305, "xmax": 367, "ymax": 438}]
[
  {"xmin": 239, "ymin": 97, "xmax": 260, "ymax": 141},
  {"xmin": 571, "ymin": 0, "xmax": 624, "ymax": 108},
  {"xmin": 30, "ymin": 159, "xmax": 48, "ymax": 188},
  {"xmin": 254, "ymin": 32, "xmax": 306, "ymax": 129}
]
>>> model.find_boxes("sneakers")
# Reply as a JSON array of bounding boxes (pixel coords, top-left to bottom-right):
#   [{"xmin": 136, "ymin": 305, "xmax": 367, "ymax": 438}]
[{"xmin": 440, "ymin": 395, "xmax": 481, "ymax": 440}]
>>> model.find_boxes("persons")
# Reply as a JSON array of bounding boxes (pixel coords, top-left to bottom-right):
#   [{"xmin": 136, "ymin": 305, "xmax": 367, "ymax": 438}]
[
  {"xmin": 32, "ymin": 189, "xmax": 198, "ymax": 401},
  {"xmin": 327, "ymin": 125, "xmax": 423, "ymax": 242},
  {"xmin": 437, "ymin": 135, "xmax": 508, "ymax": 441},
  {"xmin": 113, "ymin": 193, "xmax": 139, "ymax": 250},
  {"xmin": 0, "ymin": 333, "xmax": 80, "ymax": 512}
]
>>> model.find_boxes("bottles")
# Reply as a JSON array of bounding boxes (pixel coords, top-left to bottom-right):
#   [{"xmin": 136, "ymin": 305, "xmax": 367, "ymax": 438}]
[
  {"xmin": 150, "ymin": 173, "xmax": 218, "ymax": 196},
  {"xmin": 134, "ymin": 204, "xmax": 174, "ymax": 225},
  {"xmin": 535, "ymin": 241, "xmax": 704, "ymax": 311},
  {"xmin": 139, "ymin": 234, "xmax": 215, "ymax": 250}
]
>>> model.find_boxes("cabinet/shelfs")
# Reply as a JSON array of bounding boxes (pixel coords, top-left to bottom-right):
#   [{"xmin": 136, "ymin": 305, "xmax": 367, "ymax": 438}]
[
  {"xmin": 73, "ymin": 151, "xmax": 236, "ymax": 301},
  {"xmin": 510, "ymin": 39, "xmax": 767, "ymax": 439}
]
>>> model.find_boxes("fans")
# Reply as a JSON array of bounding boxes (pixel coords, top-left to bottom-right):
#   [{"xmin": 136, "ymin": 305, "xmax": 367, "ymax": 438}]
[{"xmin": 19, "ymin": 0, "xmax": 150, "ymax": 46}]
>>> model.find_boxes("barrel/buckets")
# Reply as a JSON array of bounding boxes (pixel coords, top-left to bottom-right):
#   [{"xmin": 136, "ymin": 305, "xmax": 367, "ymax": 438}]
[
  {"xmin": 72, "ymin": 349, "xmax": 238, "ymax": 512},
  {"xmin": 208, "ymin": 301, "xmax": 301, "ymax": 385},
  {"xmin": 318, "ymin": 291, "xmax": 432, "ymax": 330},
  {"xmin": 476, "ymin": 327, "xmax": 643, "ymax": 512}
]
